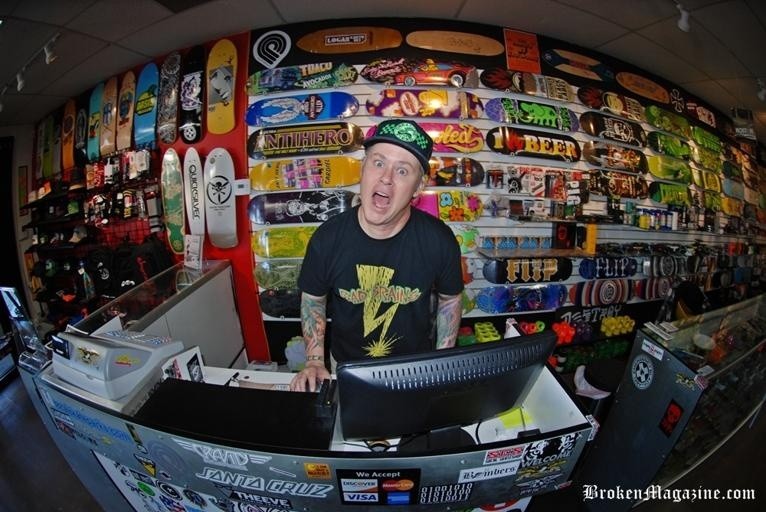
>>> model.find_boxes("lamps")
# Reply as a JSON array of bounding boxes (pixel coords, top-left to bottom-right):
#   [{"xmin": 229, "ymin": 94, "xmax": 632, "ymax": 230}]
[
  {"xmin": 0, "ymin": 31, "xmax": 73, "ymax": 113},
  {"xmin": 676, "ymin": 3, "xmax": 692, "ymax": 33}
]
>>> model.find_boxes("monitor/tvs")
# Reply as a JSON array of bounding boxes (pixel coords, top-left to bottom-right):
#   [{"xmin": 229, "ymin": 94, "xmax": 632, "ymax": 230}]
[{"xmin": 336, "ymin": 331, "xmax": 558, "ymax": 455}]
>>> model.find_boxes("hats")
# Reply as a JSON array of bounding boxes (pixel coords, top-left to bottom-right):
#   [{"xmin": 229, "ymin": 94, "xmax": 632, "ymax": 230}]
[{"xmin": 363, "ymin": 119, "xmax": 433, "ymax": 176}]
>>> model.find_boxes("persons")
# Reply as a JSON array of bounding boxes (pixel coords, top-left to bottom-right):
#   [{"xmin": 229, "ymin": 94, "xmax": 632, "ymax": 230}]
[{"xmin": 287, "ymin": 117, "xmax": 464, "ymax": 393}]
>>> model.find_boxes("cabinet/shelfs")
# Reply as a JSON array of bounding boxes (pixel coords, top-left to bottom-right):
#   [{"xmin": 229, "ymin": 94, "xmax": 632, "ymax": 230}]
[{"xmin": 20, "ymin": 196, "xmax": 99, "ymax": 324}]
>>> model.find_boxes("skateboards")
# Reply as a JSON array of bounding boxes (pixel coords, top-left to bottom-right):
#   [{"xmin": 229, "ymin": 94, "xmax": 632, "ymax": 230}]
[
  {"xmin": 161, "ymin": 146, "xmax": 238, "ymax": 254},
  {"xmin": 244, "ymin": 57, "xmax": 766, "ymax": 320},
  {"xmin": 35, "ymin": 39, "xmax": 236, "ymax": 183}
]
